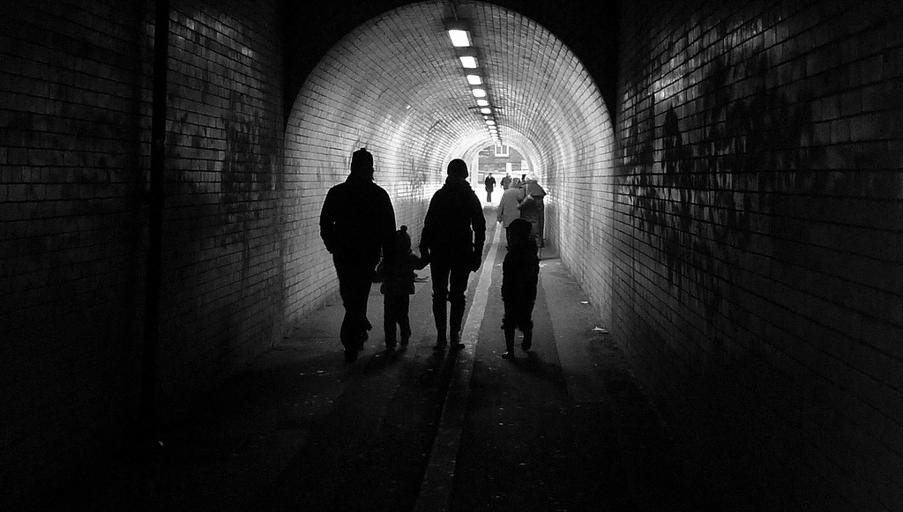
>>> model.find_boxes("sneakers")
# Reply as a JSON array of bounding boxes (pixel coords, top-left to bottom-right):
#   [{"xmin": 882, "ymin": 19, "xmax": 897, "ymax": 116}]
[{"xmin": 343, "ymin": 333, "xmax": 534, "ymax": 362}]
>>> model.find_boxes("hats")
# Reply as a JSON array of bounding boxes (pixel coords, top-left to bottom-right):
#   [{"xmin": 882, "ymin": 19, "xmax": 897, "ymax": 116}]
[
  {"xmin": 506, "ymin": 219, "xmax": 532, "ymax": 235},
  {"xmin": 447, "ymin": 160, "xmax": 469, "ymax": 178},
  {"xmin": 351, "ymin": 149, "xmax": 375, "ymax": 165},
  {"xmin": 396, "ymin": 225, "xmax": 412, "ymax": 252},
  {"xmin": 510, "ymin": 178, "xmax": 523, "ymax": 187}
]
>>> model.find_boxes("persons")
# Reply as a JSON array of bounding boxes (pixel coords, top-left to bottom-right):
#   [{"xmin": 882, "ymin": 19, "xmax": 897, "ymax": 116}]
[
  {"xmin": 417, "ymin": 156, "xmax": 487, "ymax": 359},
  {"xmin": 368, "ymin": 221, "xmax": 426, "ymax": 353},
  {"xmin": 318, "ymin": 145, "xmax": 395, "ymax": 351},
  {"xmin": 500, "ymin": 220, "xmax": 541, "ymax": 359},
  {"xmin": 477, "ymin": 168, "xmax": 547, "ymax": 223}
]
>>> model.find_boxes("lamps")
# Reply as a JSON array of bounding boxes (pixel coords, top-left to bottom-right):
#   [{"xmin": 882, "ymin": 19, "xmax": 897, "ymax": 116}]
[{"xmin": 445, "ymin": 22, "xmax": 502, "ymax": 147}]
